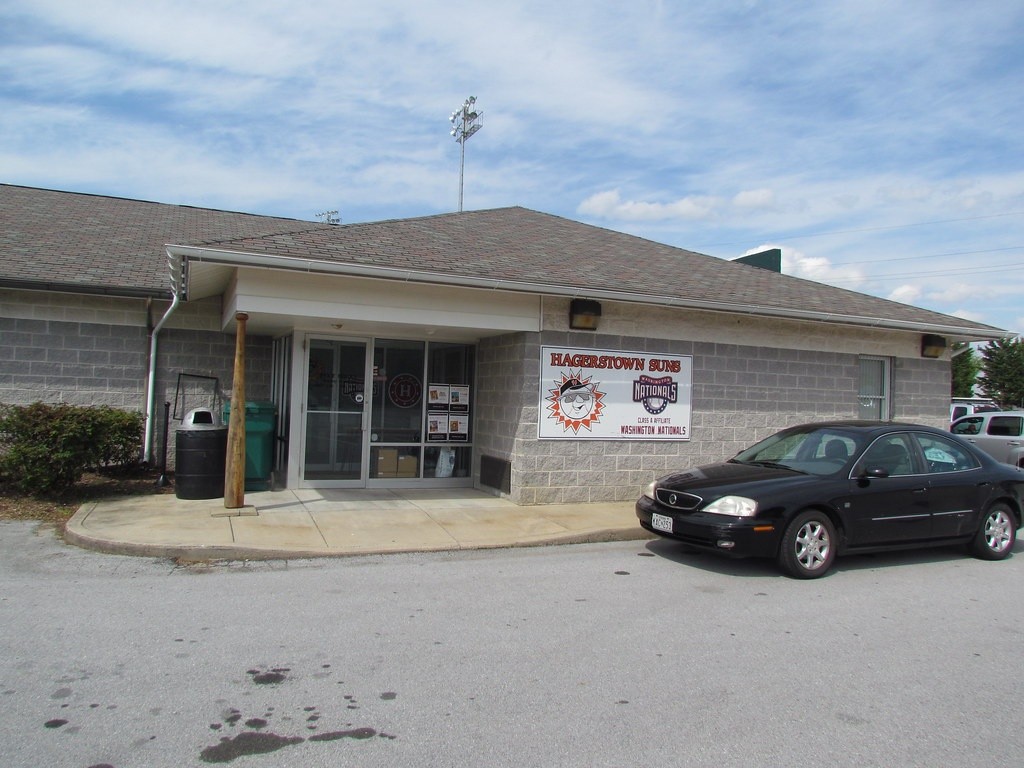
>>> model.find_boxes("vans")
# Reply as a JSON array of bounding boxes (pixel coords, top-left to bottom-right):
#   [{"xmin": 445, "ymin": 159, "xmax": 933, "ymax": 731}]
[{"xmin": 950, "ymin": 401, "xmax": 1006, "ymax": 434}]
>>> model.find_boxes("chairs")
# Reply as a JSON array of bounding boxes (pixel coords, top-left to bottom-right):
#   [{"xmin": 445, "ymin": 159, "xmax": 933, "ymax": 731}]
[{"xmin": 825, "ymin": 438, "xmax": 960, "ymax": 474}]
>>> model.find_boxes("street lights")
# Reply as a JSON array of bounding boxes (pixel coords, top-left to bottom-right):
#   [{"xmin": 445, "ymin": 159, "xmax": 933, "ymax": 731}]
[{"xmin": 449, "ymin": 94, "xmax": 484, "ymax": 213}]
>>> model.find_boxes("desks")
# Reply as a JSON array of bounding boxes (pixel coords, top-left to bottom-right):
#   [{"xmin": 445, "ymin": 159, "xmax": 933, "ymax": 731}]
[{"xmin": 340, "ymin": 428, "xmax": 420, "ymax": 473}]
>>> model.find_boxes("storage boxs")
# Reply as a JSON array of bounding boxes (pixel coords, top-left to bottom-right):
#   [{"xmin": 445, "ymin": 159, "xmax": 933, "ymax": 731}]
[{"xmin": 376, "ymin": 449, "xmax": 418, "ymax": 479}]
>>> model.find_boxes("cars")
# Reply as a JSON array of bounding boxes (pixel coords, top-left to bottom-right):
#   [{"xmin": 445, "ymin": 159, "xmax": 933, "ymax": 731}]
[
  {"xmin": 635, "ymin": 419, "xmax": 1024, "ymax": 581},
  {"xmin": 950, "ymin": 410, "xmax": 1024, "ymax": 469}
]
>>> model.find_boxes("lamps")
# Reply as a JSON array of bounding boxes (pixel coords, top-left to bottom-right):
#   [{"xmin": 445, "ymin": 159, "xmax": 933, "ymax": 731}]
[
  {"xmin": 921, "ymin": 334, "xmax": 947, "ymax": 358},
  {"xmin": 569, "ymin": 298, "xmax": 602, "ymax": 331}
]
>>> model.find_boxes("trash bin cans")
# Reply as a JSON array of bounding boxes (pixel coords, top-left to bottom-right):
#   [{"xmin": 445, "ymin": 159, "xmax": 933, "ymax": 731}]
[
  {"xmin": 223, "ymin": 400, "xmax": 276, "ymax": 491},
  {"xmin": 175, "ymin": 407, "xmax": 229, "ymax": 500}
]
